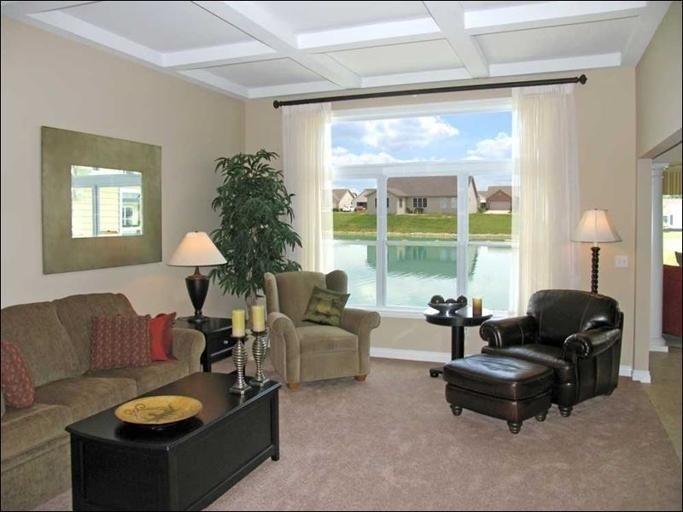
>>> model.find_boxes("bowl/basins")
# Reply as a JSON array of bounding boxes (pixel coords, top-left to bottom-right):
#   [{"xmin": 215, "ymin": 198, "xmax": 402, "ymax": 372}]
[
  {"xmin": 427, "ymin": 303, "xmax": 467, "ymax": 312},
  {"xmin": 432, "ymin": 311, "xmax": 462, "ymax": 318}
]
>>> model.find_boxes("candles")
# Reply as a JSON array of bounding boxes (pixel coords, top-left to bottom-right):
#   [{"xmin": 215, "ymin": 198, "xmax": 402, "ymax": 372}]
[
  {"xmin": 251, "ymin": 305, "xmax": 266, "ymax": 332},
  {"xmin": 230, "ymin": 309, "xmax": 246, "ymax": 338}
]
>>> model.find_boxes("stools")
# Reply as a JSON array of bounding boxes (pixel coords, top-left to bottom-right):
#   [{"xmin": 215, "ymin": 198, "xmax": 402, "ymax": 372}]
[{"xmin": 443, "ymin": 353, "xmax": 554, "ymax": 434}]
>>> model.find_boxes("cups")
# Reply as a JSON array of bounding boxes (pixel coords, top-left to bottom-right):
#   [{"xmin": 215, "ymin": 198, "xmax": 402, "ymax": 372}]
[{"xmin": 472, "ymin": 296, "xmax": 482, "ymax": 317}]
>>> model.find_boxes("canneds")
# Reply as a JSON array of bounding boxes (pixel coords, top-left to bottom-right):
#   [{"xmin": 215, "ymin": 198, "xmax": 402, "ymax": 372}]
[{"xmin": 474, "ymin": 298, "xmax": 482, "ymax": 316}]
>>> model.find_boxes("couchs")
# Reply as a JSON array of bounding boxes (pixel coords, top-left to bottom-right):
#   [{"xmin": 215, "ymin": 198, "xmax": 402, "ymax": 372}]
[{"xmin": 1, "ymin": 292, "xmax": 207, "ymax": 511}]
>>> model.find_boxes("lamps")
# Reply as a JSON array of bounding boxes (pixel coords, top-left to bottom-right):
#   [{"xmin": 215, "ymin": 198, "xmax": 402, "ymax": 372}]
[
  {"xmin": 570, "ymin": 208, "xmax": 622, "ymax": 295},
  {"xmin": 168, "ymin": 231, "xmax": 228, "ymax": 324}
]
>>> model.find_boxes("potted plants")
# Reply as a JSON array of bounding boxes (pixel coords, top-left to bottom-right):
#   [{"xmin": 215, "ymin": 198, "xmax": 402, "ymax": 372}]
[{"xmin": 208, "ymin": 148, "xmax": 303, "ymax": 363}]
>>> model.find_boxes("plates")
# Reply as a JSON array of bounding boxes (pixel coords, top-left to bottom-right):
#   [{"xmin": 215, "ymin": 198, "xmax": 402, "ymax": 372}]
[{"xmin": 110, "ymin": 394, "xmax": 204, "ymax": 432}]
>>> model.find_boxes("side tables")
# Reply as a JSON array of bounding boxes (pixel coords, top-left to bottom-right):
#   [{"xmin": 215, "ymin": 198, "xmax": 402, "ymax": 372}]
[
  {"xmin": 173, "ymin": 316, "xmax": 248, "ymax": 375},
  {"xmin": 423, "ymin": 306, "xmax": 493, "ymax": 377}
]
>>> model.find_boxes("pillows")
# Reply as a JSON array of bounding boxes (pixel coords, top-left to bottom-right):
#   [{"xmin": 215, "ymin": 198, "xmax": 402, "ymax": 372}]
[
  {"xmin": 117, "ymin": 312, "xmax": 178, "ymax": 361},
  {"xmin": 304, "ymin": 284, "xmax": 350, "ymax": 326},
  {"xmin": 89, "ymin": 314, "xmax": 151, "ymax": 372},
  {"xmin": 1, "ymin": 340, "xmax": 34, "ymax": 408}
]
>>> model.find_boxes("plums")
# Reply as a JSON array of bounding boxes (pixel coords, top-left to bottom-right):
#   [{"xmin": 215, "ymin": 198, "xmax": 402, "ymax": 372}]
[{"xmin": 432, "ymin": 296, "xmax": 466, "ymax": 303}]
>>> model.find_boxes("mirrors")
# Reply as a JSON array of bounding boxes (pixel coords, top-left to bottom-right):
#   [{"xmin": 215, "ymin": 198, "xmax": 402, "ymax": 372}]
[{"xmin": 41, "ymin": 125, "xmax": 162, "ymax": 275}]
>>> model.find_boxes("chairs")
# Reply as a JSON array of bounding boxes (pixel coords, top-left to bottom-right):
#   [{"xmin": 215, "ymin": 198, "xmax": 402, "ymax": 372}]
[
  {"xmin": 263, "ymin": 270, "xmax": 380, "ymax": 390},
  {"xmin": 480, "ymin": 289, "xmax": 623, "ymax": 417}
]
